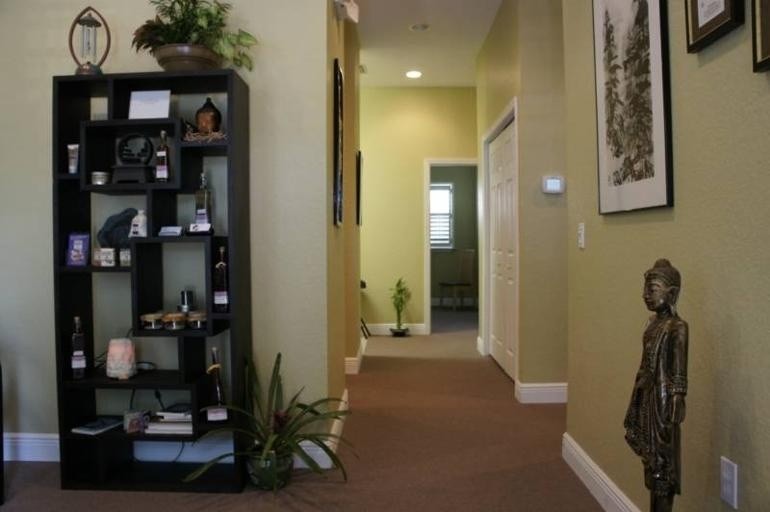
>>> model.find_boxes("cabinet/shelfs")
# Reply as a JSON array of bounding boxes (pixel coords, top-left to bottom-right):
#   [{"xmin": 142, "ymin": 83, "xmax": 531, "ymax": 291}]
[{"xmin": 49, "ymin": 69, "xmax": 254, "ymax": 495}]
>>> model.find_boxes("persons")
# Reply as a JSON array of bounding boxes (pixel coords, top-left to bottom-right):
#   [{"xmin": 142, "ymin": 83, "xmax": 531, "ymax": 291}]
[{"xmin": 623, "ymin": 258, "xmax": 688, "ymax": 512}]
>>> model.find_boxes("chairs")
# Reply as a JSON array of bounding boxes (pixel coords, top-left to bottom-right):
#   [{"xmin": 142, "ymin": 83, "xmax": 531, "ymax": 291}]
[{"xmin": 439, "ymin": 246, "xmax": 478, "ymax": 313}]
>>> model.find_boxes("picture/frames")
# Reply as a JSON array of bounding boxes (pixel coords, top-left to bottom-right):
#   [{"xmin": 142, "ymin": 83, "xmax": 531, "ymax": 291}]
[
  {"xmin": 333, "ymin": 54, "xmax": 346, "ymax": 230},
  {"xmin": 682, "ymin": 0, "xmax": 746, "ymax": 57},
  {"xmin": 750, "ymin": 1, "xmax": 770, "ymax": 74},
  {"xmin": 591, "ymin": 0, "xmax": 675, "ymax": 218}
]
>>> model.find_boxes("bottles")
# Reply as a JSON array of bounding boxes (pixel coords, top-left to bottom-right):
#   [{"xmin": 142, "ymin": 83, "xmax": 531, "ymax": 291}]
[
  {"xmin": 195, "ymin": 97, "xmax": 223, "ymax": 133},
  {"xmin": 128, "ymin": 209, "xmax": 147, "ymax": 238},
  {"xmin": 155, "ymin": 131, "xmax": 170, "ymax": 182},
  {"xmin": 141, "ymin": 309, "xmax": 207, "ymax": 331},
  {"xmin": 181, "ymin": 289, "xmax": 192, "ymax": 305},
  {"xmin": 210, "ymin": 348, "xmax": 227, "ymax": 405},
  {"xmin": 194, "ymin": 171, "xmax": 212, "ymax": 226},
  {"xmin": 91, "ymin": 171, "xmax": 110, "ymax": 185},
  {"xmin": 70, "ymin": 315, "xmax": 86, "ymax": 379},
  {"xmin": 213, "ymin": 246, "xmax": 230, "ymax": 313}
]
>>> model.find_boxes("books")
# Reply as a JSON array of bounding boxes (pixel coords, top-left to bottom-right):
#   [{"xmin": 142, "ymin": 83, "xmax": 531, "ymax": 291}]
[
  {"xmin": 72, "ymin": 417, "xmax": 123, "ymax": 435},
  {"xmin": 145, "ymin": 403, "xmax": 193, "ymax": 434}
]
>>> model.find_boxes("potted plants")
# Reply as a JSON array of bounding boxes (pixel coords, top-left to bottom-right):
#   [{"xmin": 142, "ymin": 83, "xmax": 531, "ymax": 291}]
[
  {"xmin": 129, "ymin": 1, "xmax": 260, "ymax": 75},
  {"xmin": 386, "ymin": 276, "xmax": 415, "ymax": 339},
  {"xmin": 176, "ymin": 348, "xmax": 363, "ymax": 496}
]
